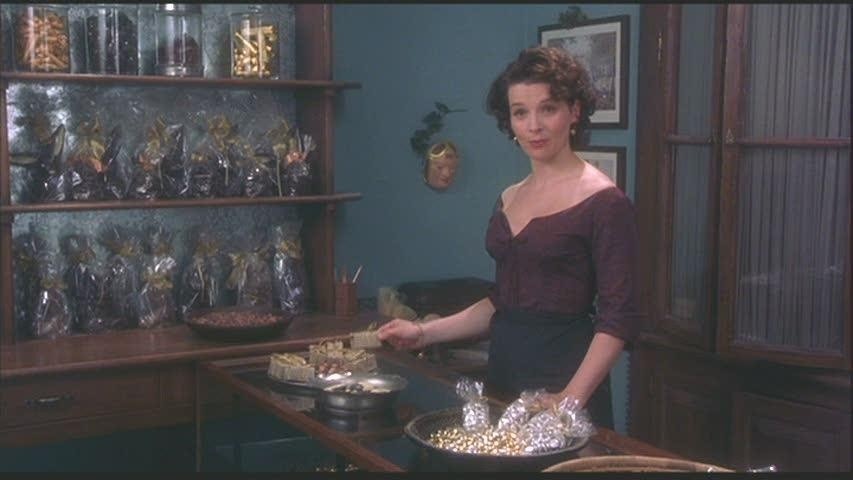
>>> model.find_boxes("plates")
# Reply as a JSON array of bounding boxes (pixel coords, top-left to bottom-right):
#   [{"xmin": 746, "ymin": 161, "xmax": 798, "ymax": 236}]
[
  {"xmin": 541, "ymin": 455, "xmax": 731, "ymax": 473},
  {"xmin": 182, "ymin": 306, "xmax": 295, "ymax": 341},
  {"xmin": 404, "ymin": 406, "xmax": 589, "ymax": 464}
]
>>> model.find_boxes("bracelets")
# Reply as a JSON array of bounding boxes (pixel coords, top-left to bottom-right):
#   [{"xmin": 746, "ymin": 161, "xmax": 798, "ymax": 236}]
[{"xmin": 414, "ymin": 322, "xmax": 424, "ymax": 358}]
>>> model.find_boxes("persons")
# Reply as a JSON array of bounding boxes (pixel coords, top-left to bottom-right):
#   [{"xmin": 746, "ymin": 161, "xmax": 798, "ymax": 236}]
[
  {"xmin": 377, "ymin": 46, "xmax": 639, "ymax": 421},
  {"xmin": 426, "ymin": 141, "xmax": 457, "ymax": 189}
]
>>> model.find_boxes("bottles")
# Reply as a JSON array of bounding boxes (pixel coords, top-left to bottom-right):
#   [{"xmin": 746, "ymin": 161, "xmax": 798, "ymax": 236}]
[{"xmin": 13, "ymin": 1, "xmax": 282, "ymax": 79}]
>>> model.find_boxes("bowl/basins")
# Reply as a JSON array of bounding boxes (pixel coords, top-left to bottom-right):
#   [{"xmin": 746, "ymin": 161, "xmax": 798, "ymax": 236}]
[{"xmin": 309, "ymin": 372, "xmax": 409, "ymax": 417}]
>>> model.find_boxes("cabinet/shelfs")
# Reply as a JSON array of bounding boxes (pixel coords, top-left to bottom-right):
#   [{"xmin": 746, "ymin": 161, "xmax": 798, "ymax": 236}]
[
  {"xmin": 623, "ymin": 0, "xmax": 852, "ymax": 480},
  {"xmin": 197, "ymin": 349, "xmax": 683, "ymax": 472},
  {"xmin": 0, "ymin": 3, "xmax": 365, "ymax": 449}
]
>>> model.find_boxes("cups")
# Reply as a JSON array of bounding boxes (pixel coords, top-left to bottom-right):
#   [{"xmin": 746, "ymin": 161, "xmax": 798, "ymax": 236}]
[{"xmin": 334, "ymin": 276, "xmax": 359, "ymax": 316}]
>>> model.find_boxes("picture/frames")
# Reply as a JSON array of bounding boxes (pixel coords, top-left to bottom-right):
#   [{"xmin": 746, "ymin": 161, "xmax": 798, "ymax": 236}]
[
  {"xmin": 569, "ymin": 144, "xmax": 627, "ymax": 197},
  {"xmin": 537, "ymin": 14, "xmax": 631, "ymax": 131}
]
